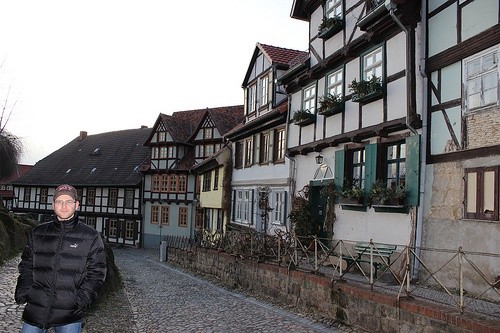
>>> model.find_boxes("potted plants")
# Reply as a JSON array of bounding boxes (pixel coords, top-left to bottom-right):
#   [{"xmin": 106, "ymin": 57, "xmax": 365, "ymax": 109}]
[
  {"xmin": 368, "ymin": 177, "xmax": 409, "ymax": 209},
  {"xmin": 331, "ymin": 178, "xmax": 366, "ymax": 204}
]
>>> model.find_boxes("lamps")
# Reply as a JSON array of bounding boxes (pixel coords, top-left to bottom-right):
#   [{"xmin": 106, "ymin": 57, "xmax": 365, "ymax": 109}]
[{"xmin": 315, "ymin": 152, "xmax": 323, "ymax": 164}]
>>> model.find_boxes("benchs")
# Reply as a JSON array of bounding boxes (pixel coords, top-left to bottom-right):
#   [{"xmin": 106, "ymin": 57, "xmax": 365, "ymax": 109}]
[{"xmin": 342, "ymin": 241, "xmax": 397, "ymax": 279}]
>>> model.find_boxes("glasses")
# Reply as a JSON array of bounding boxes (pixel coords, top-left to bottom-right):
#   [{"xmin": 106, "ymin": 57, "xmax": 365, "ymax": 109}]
[{"xmin": 54, "ymin": 200, "xmax": 76, "ymax": 205}]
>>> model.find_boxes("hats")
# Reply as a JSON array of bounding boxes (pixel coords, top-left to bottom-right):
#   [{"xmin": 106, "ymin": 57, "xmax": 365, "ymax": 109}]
[{"xmin": 53, "ymin": 184, "xmax": 79, "ymax": 202}]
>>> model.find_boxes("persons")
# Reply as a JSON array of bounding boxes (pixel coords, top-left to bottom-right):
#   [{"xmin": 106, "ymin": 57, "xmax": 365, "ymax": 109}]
[{"xmin": 14, "ymin": 184, "xmax": 107, "ymax": 333}]
